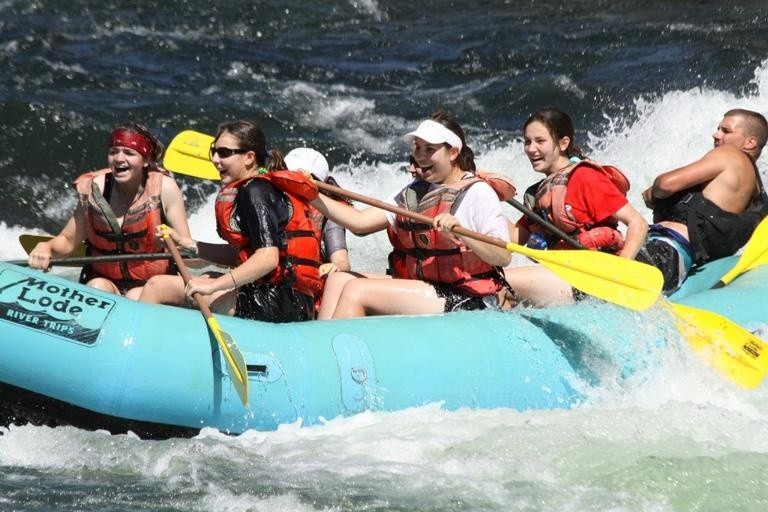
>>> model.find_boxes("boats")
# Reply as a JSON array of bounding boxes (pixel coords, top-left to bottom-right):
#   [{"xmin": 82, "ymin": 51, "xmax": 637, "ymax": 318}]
[{"xmin": 1, "ymin": 252, "xmax": 766, "ymax": 434}]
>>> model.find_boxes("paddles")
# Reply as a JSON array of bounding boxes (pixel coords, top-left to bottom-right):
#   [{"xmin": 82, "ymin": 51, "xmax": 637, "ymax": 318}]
[
  {"xmin": 19, "ymin": 235, "xmax": 88, "ymax": 258},
  {"xmin": 163, "ymin": 131, "xmax": 665, "ymax": 312},
  {"xmin": 708, "ymin": 215, "xmax": 768, "ymax": 289},
  {"xmin": 160, "ymin": 224, "xmax": 249, "ymax": 405},
  {"xmin": 506, "ymin": 198, "xmax": 768, "ymax": 389}
]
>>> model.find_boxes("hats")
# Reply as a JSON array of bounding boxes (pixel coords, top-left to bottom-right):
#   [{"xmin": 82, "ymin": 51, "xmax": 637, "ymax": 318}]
[
  {"xmin": 403, "ymin": 119, "xmax": 464, "ymax": 156},
  {"xmin": 282, "ymin": 147, "xmax": 330, "ymax": 183}
]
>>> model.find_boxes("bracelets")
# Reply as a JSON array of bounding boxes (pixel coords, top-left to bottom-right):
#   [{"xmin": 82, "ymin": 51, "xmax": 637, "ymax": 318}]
[{"xmin": 229, "ymin": 268, "xmax": 238, "ymax": 289}]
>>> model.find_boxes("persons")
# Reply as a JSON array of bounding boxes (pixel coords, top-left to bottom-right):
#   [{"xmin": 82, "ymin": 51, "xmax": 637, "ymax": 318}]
[
  {"xmin": 635, "ymin": 109, "xmax": 768, "ymax": 296},
  {"xmin": 297, "ymin": 111, "xmax": 511, "ymax": 319},
  {"xmin": 138, "ymin": 122, "xmax": 320, "ymax": 324},
  {"xmin": 499, "ymin": 108, "xmax": 648, "ymax": 308},
  {"xmin": 410, "ymin": 145, "xmax": 424, "ymax": 180},
  {"xmin": 269, "ymin": 147, "xmax": 353, "ymax": 277},
  {"xmin": 28, "ymin": 123, "xmax": 191, "ymax": 301}
]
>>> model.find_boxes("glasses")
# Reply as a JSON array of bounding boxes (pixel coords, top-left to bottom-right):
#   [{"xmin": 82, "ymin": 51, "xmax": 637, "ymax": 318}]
[{"xmin": 210, "ymin": 146, "xmax": 248, "ymax": 158}]
